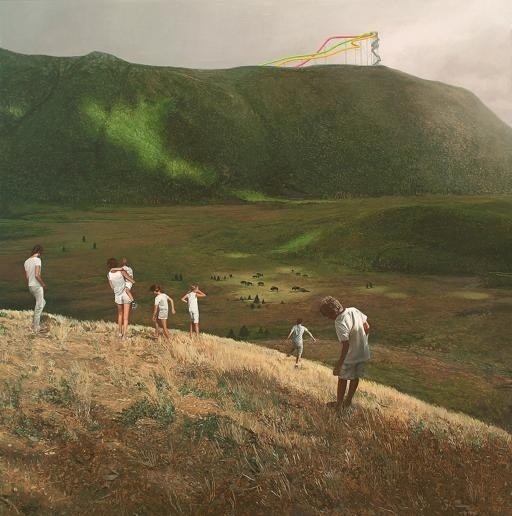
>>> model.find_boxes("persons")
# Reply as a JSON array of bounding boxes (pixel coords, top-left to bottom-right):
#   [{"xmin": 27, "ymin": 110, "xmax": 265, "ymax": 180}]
[
  {"xmin": 285, "ymin": 318, "xmax": 316, "ymax": 367},
  {"xmin": 111, "ymin": 258, "xmax": 138, "ymax": 309},
  {"xmin": 23, "ymin": 245, "xmax": 48, "ymax": 333},
  {"xmin": 320, "ymin": 295, "xmax": 371, "ymax": 410},
  {"xmin": 151, "ymin": 284, "xmax": 176, "ymax": 337},
  {"xmin": 180, "ymin": 281, "xmax": 207, "ymax": 337},
  {"xmin": 106, "ymin": 258, "xmax": 136, "ymax": 341}
]
